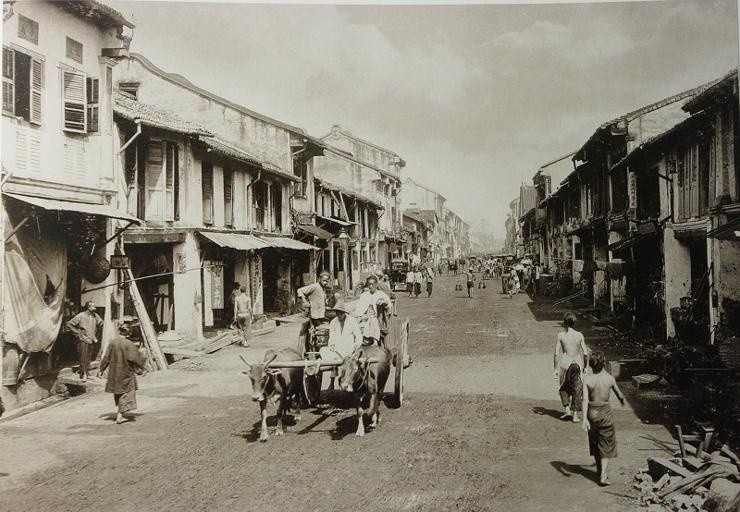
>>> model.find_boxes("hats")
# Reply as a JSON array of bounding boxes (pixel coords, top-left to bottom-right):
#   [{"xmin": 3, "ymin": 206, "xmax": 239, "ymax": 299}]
[{"xmin": 326, "ymin": 301, "xmax": 349, "ymax": 314}]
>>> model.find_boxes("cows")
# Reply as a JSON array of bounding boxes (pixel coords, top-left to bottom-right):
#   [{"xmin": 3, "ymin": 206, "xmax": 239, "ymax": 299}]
[
  {"xmin": 337, "ymin": 343, "xmax": 390, "ymax": 438},
  {"xmin": 238, "ymin": 345, "xmax": 304, "ymax": 443}
]
